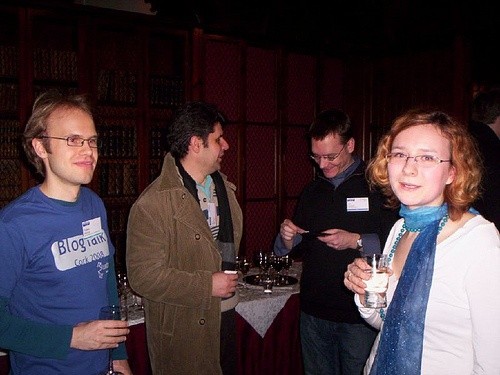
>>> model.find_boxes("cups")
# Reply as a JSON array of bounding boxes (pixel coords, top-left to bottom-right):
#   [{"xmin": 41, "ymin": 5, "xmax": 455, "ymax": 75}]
[
  {"xmin": 359, "ymin": 254, "xmax": 391, "ymax": 308},
  {"xmin": 262, "ymin": 275, "xmax": 273, "ymax": 294}
]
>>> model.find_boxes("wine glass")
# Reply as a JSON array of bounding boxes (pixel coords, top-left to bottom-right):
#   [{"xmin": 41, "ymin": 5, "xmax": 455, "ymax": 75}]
[
  {"xmin": 99, "ymin": 304, "xmax": 127, "ymax": 375},
  {"xmin": 234, "ymin": 250, "xmax": 294, "ymax": 285},
  {"xmin": 114, "ymin": 270, "xmax": 146, "ymax": 313}
]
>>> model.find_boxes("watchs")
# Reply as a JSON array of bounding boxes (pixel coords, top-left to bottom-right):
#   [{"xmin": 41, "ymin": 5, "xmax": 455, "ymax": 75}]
[{"xmin": 355, "ymin": 234, "xmax": 363, "ymax": 250}]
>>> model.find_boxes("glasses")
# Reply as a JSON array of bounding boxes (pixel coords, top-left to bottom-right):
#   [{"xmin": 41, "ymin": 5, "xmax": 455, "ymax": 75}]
[
  {"xmin": 311, "ymin": 137, "xmax": 349, "ymax": 161},
  {"xmin": 34, "ymin": 135, "xmax": 102, "ymax": 148},
  {"xmin": 386, "ymin": 153, "xmax": 452, "ymax": 166}
]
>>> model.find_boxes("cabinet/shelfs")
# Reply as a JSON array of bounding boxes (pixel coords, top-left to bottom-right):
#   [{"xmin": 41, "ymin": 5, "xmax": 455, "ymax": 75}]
[{"xmin": 0, "ymin": 0, "xmax": 205, "ymax": 267}]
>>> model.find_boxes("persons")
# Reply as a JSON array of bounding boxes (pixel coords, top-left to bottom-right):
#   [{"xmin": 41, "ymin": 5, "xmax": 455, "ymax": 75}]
[
  {"xmin": 272, "ymin": 108, "xmax": 402, "ymax": 374},
  {"xmin": 346, "ymin": 87, "xmax": 500, "ymax": 375},
  {"xmin": 0, "ymin": 88, "xmax": 133, "ymax": 374},
  {"xmin": 127, "ymin": 101, "xmax": 249, "ymax": 375}
]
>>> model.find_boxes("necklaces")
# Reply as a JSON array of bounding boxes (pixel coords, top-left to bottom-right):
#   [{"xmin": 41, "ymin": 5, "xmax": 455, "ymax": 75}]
[{"xmin": 380, "ymin": 208, "xmax": 449, "ymax": 326}]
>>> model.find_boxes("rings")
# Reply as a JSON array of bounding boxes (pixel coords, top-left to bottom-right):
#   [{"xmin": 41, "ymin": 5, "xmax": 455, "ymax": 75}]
[{"xmin": 347, "ymin": 273, "xmax": 353, "ymax": 282}]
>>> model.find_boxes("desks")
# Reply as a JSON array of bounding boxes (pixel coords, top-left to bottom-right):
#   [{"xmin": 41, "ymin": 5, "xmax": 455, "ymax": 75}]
[{"xmin": 115, "ymin": 260, "xmax": 304, "ymax": 375}]
